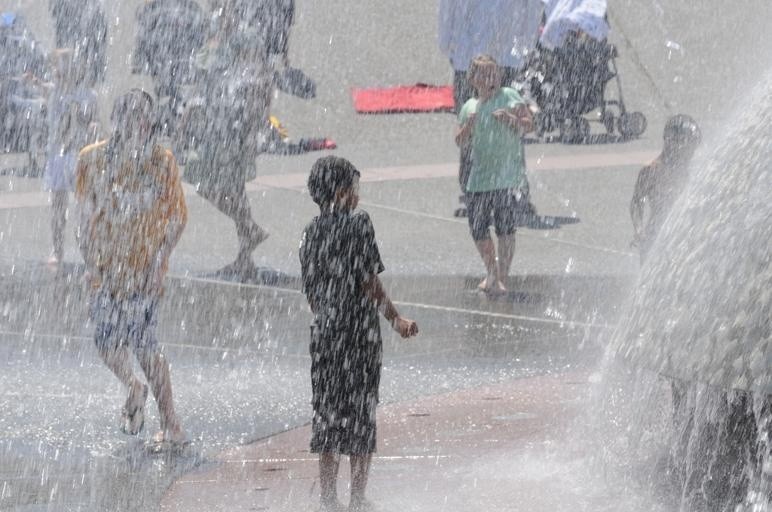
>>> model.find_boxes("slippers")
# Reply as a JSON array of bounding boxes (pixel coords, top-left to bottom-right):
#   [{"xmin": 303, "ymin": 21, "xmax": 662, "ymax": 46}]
[
  {"xmin": 118, "ymin": 384, "xmax": 148, "ymax": 435},
  {"xmin": 150, "ymin": 439, "xmax": 193, "ymax": 454}
]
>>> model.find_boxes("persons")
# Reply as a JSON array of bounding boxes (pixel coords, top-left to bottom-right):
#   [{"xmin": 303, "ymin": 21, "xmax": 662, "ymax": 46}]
[
  {"xmin": 438, "ymin": 1, "xmax": 547, "ymax": 220},
  {"xmin": 655, "ymin": 386, "xmax": 768, "ymax": 512},
  {"xmin": 630, "ymin": 115, "xmax": 700, "ymax": 323},
  {"xmin": 455, "ymin": 53, "xmax": 533, "ymax": 296},
  {"xmin": 298, "ymin": 153, "xmax": 418, "ymax": 512},
  {"xmin": 2, "ymin": 0, "xmax": 293, "ymax": 275},
  {"xmin": 74, "ymin": 90, "xmax": 188, "ymax": 453}
]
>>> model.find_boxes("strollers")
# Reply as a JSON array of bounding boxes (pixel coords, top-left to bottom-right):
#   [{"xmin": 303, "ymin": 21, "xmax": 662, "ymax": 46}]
[{"xmin": 518, "ymin": 0, "xmax": 647, "ymax": 143}]
